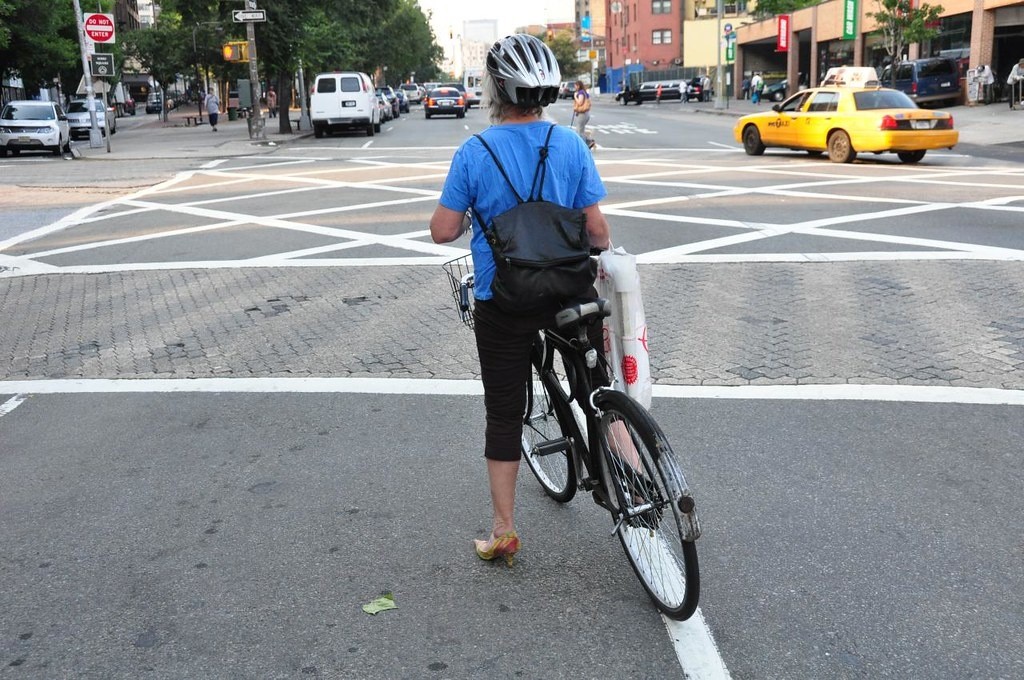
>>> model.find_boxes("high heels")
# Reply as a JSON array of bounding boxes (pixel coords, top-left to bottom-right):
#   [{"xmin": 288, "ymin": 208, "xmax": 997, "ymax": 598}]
[
  {"xmin": 474, "ymin": 532, "xmax": 522, "ymax": 568},
  {"xmin": 626, "ymin": 514, "xmax": 660, "ymax": 538}
]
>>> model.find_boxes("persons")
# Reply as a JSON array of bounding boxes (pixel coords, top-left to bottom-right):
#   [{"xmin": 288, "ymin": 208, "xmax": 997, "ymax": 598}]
[
  {"xmin": 742, "ymin": 77, "xmax": 751, "ymax": 99},
  {"xmin": 702, "ymin": 75, "xmax": 711, "ymax": 102},
  {"xmin": 204, "ymin": 87, "xmax": 219, "ymax": 132},
  {"xmin": 429, "ymin": 33, "xmax": 642, "ymax": 566},
  {"xmin": 1007, "ymin": 58, "xmax": 1024, "ymax": 110},
  {"xmin": 267, "ymin": 86, "xmax": 277, "ymax": 118},
  {"xmin": 976, "ymin": 65, "xmax": 994, "ymax": 105},
  {"xmin": 751, "ymin": 71, "xmax": 763, "ymax": 102},
  {"xmin": 679, "ymin": 79, "xmax": 688, "ymax": 103},
  {"xmin": 573, "ymin": 80, "xmax": 594, "ymax": 150}
]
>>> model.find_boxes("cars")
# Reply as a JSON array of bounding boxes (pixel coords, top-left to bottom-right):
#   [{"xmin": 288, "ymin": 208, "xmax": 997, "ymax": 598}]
[
  {"xmin": 615, "ymin": 79, "xmax": 715, "ymax": 105},
  {"xmin": 761, "ymin": 72, "xmax": 810, "ymax": 102},
  {"xmin": 394, "ymin": 88, "xmax": 410, "ymax": 113},
  {"xmin": 419, "ymin": 85, "xmax": 426, "ymax": 100},
  {"xmin": 559, "ymin": 81, "xmax": 576, "ymax": 99},
  {"xmin": 734, "ymin": 80, "xmax": 959, "ymax": 163},
  {"xmin": 376, "ymin": 92, "xmax": 393, "ymax": 123},
  {"xmin": 0, "ymin": 100, "xmax": 72, "ymax": 158},
  {"xmin": 424, "ymin": 86, "xmax": 465, "ymax": 119},
  {"xmin": 123, "ymin": 98, "xmax": 135, "ymax": 115},
  {"xmin": 64, "ymin": 99, "xmax": 116, "ymax": 139}
]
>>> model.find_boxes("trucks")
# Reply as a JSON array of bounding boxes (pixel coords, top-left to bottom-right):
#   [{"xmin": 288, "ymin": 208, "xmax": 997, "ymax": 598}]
[
  {"xmin": 463, "ymin": 67, "xmax": 484, "ymax": 108},
  {"xmin": 75, "ymin": 74, "xmax": 124, "ymax": 117}
]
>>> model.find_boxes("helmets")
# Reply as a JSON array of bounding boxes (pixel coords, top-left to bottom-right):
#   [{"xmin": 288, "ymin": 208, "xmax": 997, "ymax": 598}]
[{"xmin": 485, "ymin": 33, "xmax": 563, "ymax": 108}]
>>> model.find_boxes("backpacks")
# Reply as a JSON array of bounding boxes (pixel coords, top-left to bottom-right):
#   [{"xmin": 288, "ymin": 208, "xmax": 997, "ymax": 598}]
[{"xmin": 470, "ymin": 124, "xmax": 598, "ymax": 318}]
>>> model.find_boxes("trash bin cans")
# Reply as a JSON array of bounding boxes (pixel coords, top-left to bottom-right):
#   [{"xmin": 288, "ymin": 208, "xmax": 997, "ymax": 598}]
[{"xmin": 227, "ymin": 107, "xmax": 238, "ymax": 121}]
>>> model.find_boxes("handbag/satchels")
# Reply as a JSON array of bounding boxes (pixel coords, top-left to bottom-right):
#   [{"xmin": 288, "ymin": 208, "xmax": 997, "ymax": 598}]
[
  {"xmin": 752, "ymin": 94, "xmax": 758, "ymax": 104},
  {"xmin": 573, "ymin": 92, "xmax": 592, "ymax": 113},
  {"xmin": 597, "ymin": 238, "xmax": 651, "ymax": 412}
]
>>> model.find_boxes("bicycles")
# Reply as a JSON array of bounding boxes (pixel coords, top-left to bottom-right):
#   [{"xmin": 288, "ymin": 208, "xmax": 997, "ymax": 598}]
[{"xmin": 442, "ymin": 252, "xmax": 703, "ymax": 621}]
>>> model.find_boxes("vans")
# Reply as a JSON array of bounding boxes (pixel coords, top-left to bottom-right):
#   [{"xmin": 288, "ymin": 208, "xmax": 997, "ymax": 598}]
[
  {"xmin": 310, "ymin": 72, "xmax": 381, "ymax": 138},
  {"xmin": 820, "ymin": 56, "xmax": 960, "ymax": 107},
  {"xmin": 145, "ymin": 89, "xmax": 183, "ymax": 114}
]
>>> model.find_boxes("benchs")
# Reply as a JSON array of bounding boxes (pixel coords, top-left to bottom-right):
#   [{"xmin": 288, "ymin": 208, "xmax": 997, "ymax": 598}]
[
  {"xmin": 184, "ymin": 116, "xmax": 200, "ymax": 125},
  {"xmin": 292, "ymin": 119, "xmax": 300, "ymax": 129}
]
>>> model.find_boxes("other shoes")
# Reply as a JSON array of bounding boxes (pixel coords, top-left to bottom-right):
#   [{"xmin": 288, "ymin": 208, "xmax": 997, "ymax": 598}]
[
  {"xmin": 212, "ymin": 128, "xmax": 217, "ymax": 132},
  {"xmin": 588, "ymin": 142, "xmax": 596, "ymax": 148},
  {"xmin": 1011, "ymin": 106, "xmax": 1015, "ymax": 109}
]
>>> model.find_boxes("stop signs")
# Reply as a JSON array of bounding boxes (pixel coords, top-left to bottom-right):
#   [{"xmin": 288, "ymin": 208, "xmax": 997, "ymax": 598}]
[{"xmin": 84, "ymin": 13, "xmax": 115, "ymax": 44}]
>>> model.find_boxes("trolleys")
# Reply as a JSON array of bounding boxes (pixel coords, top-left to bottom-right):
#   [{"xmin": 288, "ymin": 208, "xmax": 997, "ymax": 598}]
[{"xmin": 1011, "ymin": 78, "xmax": 1024, "ymax": 110}]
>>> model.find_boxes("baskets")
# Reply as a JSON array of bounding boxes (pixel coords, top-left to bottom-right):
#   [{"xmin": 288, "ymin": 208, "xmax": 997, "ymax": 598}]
[{"xmin": 442, "ymin": 253, "xmax": 475, "ymax": 330}]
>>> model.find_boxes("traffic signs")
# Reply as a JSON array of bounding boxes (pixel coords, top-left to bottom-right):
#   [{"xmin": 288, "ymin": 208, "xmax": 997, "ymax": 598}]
[{"xmin": 232, "ymin": 10, "xmax": 266, "ymax": 23}]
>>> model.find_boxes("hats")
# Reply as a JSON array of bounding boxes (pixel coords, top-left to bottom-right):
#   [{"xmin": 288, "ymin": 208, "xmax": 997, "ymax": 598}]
[{"xmin": 1019, "ymin": 59, "xmax": 1024, "ymax": 65}]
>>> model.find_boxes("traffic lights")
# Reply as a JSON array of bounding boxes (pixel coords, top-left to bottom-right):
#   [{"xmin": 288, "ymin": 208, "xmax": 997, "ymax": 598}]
[
  {"xmin": 450, "ymin": 33, "xmax": 453, "ymax": 39},
  {"xmin": 224, "ymin": 44, "xmax": 239, "ymax": 61},
  {"xmin": 547, "ymin": 30, "xmax": 553, "ymax": 41}
]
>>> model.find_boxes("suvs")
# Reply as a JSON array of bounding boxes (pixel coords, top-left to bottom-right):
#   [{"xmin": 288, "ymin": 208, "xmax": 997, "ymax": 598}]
[
  {"xmin": 441, "ymin": 83, "xmax": 468, "ymax": 112},
  {"xmin": 399, "ymin": 83, "xmax": 421, "ymax": 104},
  {"xmin": 375, "ymin": 85, "xmax": 400, "ymax": 118}
]
